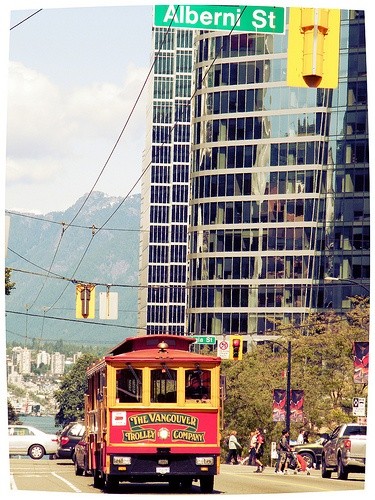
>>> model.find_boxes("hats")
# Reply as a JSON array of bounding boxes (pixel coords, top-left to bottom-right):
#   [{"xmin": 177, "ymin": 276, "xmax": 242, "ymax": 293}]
[{"xmin": 230, "ymin": 430, "xmax": 237, "ymax": 436}]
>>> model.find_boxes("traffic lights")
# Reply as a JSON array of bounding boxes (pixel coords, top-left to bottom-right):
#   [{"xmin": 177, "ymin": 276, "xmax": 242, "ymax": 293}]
[{"xmin": 228, "ymin": 336, "xmax": 243, "ymax": 362}]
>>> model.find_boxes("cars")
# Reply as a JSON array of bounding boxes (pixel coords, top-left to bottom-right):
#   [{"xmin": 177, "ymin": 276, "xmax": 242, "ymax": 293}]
[{"xmin": 7, "ymin": 425, "xmax": 60, "ymax": 460}]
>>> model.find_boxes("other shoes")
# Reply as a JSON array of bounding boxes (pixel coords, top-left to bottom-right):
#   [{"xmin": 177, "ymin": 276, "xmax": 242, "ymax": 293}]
[
  {"xmin": 262, "ymin": 464, "xmax": 266, "ymax": 472},
  {"xmin": 275, "ymin": 470, "xmax": 284, "ymax": 475},
  {"xmin": 253, "ymin": 470, "xmax": 261, "ymax": 474}
]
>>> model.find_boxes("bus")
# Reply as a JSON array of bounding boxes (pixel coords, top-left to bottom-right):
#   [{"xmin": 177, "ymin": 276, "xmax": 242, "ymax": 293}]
[{"xmin": 84, "ymin": 334, "xmax": 228, "ymax": 494}]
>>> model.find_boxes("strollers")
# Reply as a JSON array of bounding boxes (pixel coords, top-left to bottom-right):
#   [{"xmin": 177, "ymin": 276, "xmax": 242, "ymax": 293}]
[{"xmin": 292, "ymin": 451, "xmax": 311, "ymax": 476}]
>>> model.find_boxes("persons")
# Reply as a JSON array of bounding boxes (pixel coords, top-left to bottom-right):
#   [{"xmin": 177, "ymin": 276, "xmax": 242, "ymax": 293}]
[
  {"xmin": 249, "ymin": 426, "xmax": 267, "ymax": 473},
  {"xmin": 185, "ymin": 375, "xmax": 208, "ymax": 399},
  {"xmin": 56, "ymin": 430, "xmax": 61, "ymax": 435},
  {"xmin": 297, "ymin": 430, "xmax": 311, "ymax": 444},
  {"xmin": 226, "ymin": 430, "xmax": 243, "ymax": 464},
  {"xmin": 275, "ymin": 429, "xmax": 307, "ymax": 475},
  {"xmin": 314, "ymin": 433, "xmax": 324, "ymax": 470}
]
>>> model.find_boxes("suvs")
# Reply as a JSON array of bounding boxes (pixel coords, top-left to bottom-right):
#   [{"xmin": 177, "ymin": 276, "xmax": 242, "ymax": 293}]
[
  {"xmin": 320, "ymin": 422, "xmax": 367, "ymax": 481},
  {"xmin": 56, "ymin": 420, "xmax": 86, "ymax": 462},
  {"xmin": 290, "ymin": 436, "xmax": 328, "ymax": 469}
]
